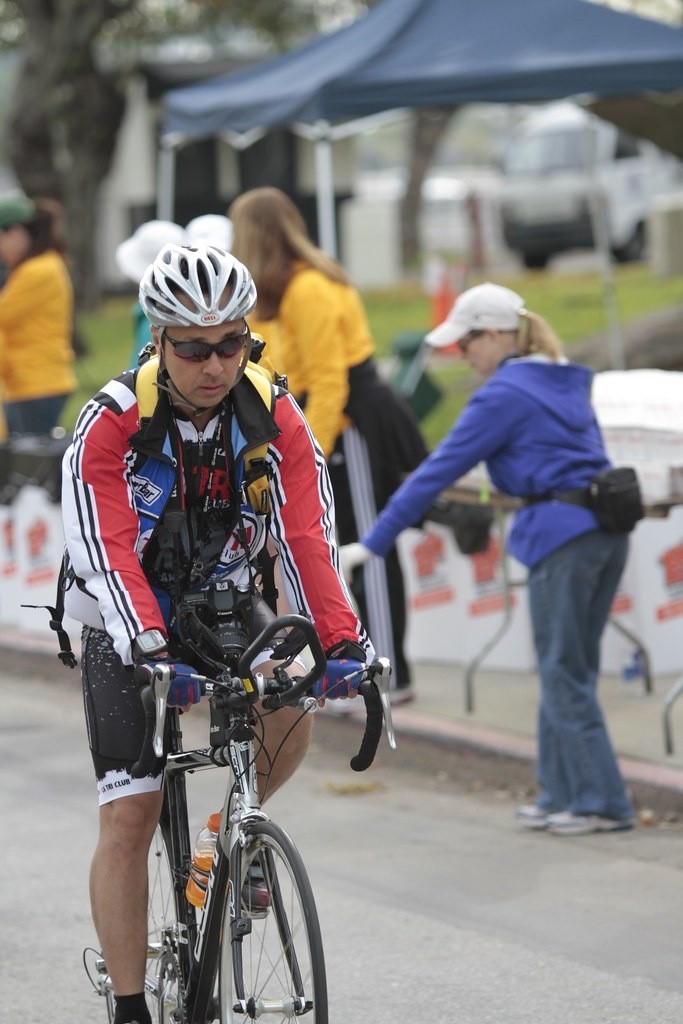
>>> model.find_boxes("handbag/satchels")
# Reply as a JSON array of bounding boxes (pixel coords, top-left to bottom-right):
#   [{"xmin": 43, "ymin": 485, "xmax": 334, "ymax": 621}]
[{"xmin": 587, "ymin": 467, "xmax": 645, "ymax": 538}]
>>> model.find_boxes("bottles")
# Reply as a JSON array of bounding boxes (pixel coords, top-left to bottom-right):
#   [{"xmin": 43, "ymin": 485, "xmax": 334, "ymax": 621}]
[{"xmin": 185, "ymin": 814, "xmax": 222, "ymax": 907}]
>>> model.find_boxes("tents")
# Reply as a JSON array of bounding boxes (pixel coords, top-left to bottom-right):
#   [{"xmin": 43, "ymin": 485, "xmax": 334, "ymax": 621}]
[{"xmin": 163, "ymin": 0, "xmax": 683, "ymax": 266}]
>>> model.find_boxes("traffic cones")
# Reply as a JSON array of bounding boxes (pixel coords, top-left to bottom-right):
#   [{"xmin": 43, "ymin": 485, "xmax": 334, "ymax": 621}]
[{"xmin": 426, "ymin": 274, "xmax": 465, "ymax": 360}]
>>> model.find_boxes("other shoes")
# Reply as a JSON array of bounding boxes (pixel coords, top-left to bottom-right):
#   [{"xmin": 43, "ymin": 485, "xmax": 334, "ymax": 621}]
[
  {"xmin": 235, "ymin": 849, "xmax": 272, "ymax": 919},
  {"xmin": 516, "ymin": 804, "xmax": 634, "ymax": 837}
]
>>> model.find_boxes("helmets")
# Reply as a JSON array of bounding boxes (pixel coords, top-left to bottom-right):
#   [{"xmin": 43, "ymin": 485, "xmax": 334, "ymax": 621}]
[{"xmin": 139, "ymin": 240, "xmax": 257, "ymax": 328}]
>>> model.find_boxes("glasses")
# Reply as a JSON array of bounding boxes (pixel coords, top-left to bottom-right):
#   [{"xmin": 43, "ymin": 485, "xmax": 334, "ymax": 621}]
[
  {"xmin": 151, "ymin": 318, "xmax": 252, "ymax": 363},
  {"xmin": 458, "ymin": 332, "xmax": 481, "ymax": 354}
]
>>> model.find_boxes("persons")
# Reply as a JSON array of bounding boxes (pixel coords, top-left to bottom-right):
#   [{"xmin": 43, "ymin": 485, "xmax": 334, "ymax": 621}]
[
  {"xmin": 0, "ymin": 188, "xmax": 427, "ymax": 715},
  {"xmin": 60, "ymin": 245, "xmax": 374, "ymax": 1024},
  {"xmin": 342, "ymin": 280, "xmax": 635, "ymax": 836}
]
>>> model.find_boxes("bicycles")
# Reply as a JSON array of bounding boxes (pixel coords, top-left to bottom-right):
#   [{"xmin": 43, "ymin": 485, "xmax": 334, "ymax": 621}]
[{"xmin": 82, "ymin": 612, "xmax": 399, "ymax": 1024}]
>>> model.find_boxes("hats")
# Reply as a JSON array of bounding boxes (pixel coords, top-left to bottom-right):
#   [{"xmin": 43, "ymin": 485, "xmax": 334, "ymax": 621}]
[
  {"xmin": 0, "ymin": 189, "xmax": 37, "ymax": 228},
  {"xmin": 114, "ymin": 221, "xmax": 187, "ymax": 285},
  {"xmin": 423, "ymin": 280, "xmax": 526, "ymax": 347},
  {"xmin": 171, "ymin": 213, "xmax": 234, "ymax": 255}
]
